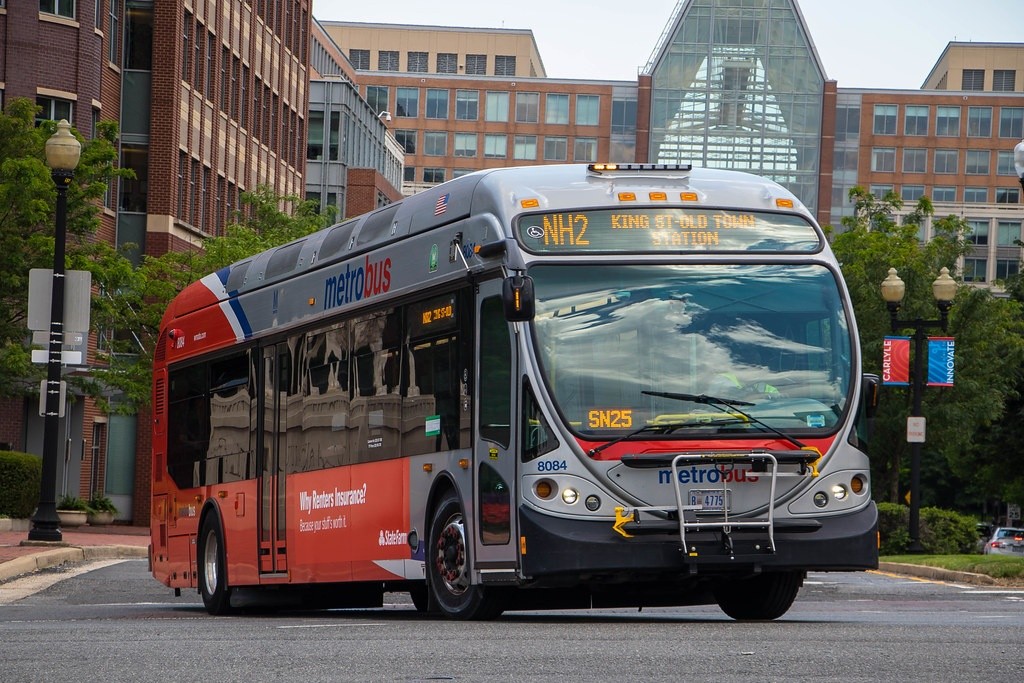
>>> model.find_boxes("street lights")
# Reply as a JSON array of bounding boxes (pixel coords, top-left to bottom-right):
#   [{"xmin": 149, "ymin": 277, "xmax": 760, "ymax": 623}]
[
  {"xmin": 882, "ymin": 266, "xmax": 958, "ymax": 554},
  {"xmin": 20, "ymin": 118, "xmax": 70, "ymax": 547}
]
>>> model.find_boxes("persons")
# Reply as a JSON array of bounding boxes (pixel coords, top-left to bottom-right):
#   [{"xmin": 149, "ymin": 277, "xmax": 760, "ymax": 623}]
[
  {"xmin": 710, "ymin": 339, "xmax": 780, "ymax": 401},
  {"xmin": 178, "ymin": 416, "xmax": 203, "ymax": 451}
]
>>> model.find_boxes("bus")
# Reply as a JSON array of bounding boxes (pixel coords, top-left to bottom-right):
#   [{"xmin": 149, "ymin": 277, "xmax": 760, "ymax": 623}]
[{"xmin": 148, "ymin": 162, "xmax": 879, "ymax": 621}]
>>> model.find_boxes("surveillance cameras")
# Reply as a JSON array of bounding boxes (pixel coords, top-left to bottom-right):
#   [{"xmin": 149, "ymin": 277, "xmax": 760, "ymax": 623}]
[{"xmin": 386, "ymin": 115, "xmax": 392, "ymax": 121}]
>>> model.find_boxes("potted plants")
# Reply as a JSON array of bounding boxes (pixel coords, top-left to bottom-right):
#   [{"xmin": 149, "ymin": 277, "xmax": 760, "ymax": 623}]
[
  {"xmin": 84, "ymin": 490, "xmax": 123, "ymax": 525},
  {"xmin": 56, "ymin": 495, "xmax": 95, "ymax": 527}
]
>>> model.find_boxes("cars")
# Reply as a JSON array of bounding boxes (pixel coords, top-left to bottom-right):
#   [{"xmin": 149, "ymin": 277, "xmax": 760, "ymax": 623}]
[
  {"xmin": 976, "ymin": 523, "xmax": 992, "ymax": 553},
  {"xmin": 984, "ymin": 527, "xmax": 1024, "ymax": 556}
]
